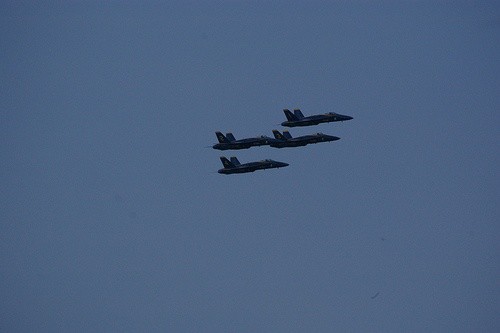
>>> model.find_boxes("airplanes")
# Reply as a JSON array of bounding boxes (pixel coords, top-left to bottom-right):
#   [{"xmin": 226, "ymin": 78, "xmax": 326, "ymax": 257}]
[
  {"xmin": 271, "ymin": 129, "xmax": 342, "ymax": 147},
  {"xmin": 218, "ymin": 155, "xmax": 290, "ymax": 174},
  {"xmin": 212, "ymin": 129, "xmax": 271, "ymax": 150},
  {"xmin": 280, "ymin": 108, "xmax": 354, "ymax": 125}
]
